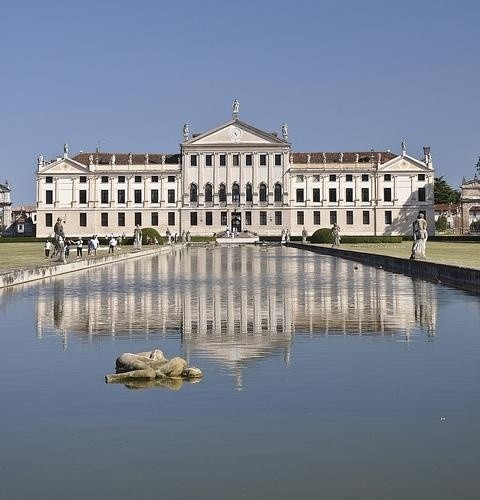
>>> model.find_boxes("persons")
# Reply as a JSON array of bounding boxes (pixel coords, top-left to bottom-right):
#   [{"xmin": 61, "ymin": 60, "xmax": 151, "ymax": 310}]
[
  {"xmin": 87, "ymin": 231, "xmax": 126, "ymax": 258},
  {"xmin": 286, "ymin": 229, "xmax": 291, "ymax": 241},
  {"xmin": 133, "ymin": 224, "xmax": 143, "ymax": 249},
  {"xmin": 412, "ymin": 213, "xmax": 428, "ymax": 230},
  {"xmin": 44, "ymin": 240, "xmax": 51, "ymax": 259},
  {"xmin": 281, "ymin": 227, "xmax": 286, "ymax": 242},
  {"xmin": 146, "ymin": 227, "xmax": 193, "ymax": 247},
  {"xmin": 50, "ymin": 238, "xmax": 59, "ymax": 258},
  {"xmin": 64, "ymin": 237, "xmax": 72, "ymax": 258},
  {"xmin": 54, "ymin": 216, "xmax": 68, "ymax": 264},
  {"xmin": 331, "ymin": 222, "xmax": 341, "ymax": 246},
  {"xmin": 301, "ymin": 227, "xmax": 308, "ymax": 243},
  {"xmin": 70, "ymin": 236, "xmax": 84, "ymax": 259},
  {"xmin": 225, "ymin": 226, "xmax": 240, "ymax": 241},
  {"xmin": 106, "ymin": 349, "xmax": 203, "ymax": 386},
  {"xmin": 411, "ymin": 222, "xmax": 428, "ymax": 259}
]
